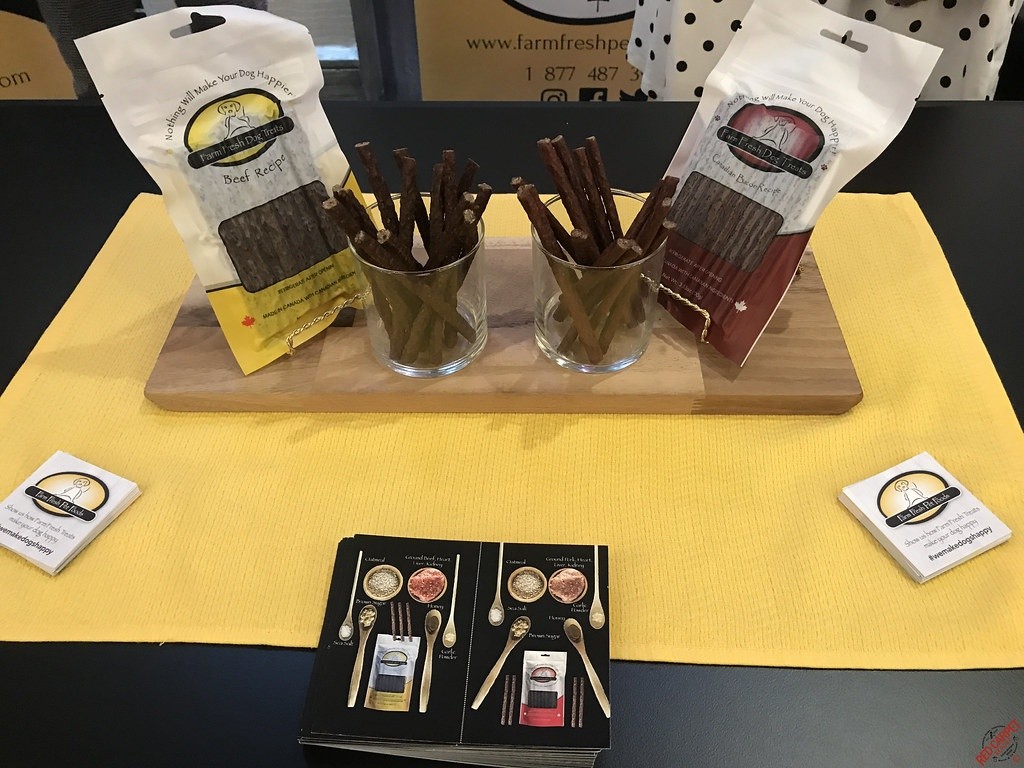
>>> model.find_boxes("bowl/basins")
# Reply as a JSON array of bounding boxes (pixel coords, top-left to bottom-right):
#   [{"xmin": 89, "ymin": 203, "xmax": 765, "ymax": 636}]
[
  {"xmin": 548, "ymin": 568, "xmax": 588, "ymax": 604},
  {"xmin": 408, "ymin": 568, "xmax": 447, "ymax": 604},
  {"xmin": 363, "ymin": 564, "xmax": 403, "ymax": 601},
  {"xmin": 507, "ymin": 567, "xmax": 547, "ymax": 603}
]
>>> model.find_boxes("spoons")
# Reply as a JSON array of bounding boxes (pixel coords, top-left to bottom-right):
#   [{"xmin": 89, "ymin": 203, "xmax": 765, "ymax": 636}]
[
  {"xmin": 488, "ymin": 541, "xmax": 505, "ymax": 627},
  {"xmin": 588, "ymin": 545, "xmax": 606, "ymax": 630},
  {"xmin": 338, "ymin": 550, "xmax": 363, "ymax": 641},
  {"xmin": 418, "ymin": 608, "xmax": 442, "ymax": 713},
  {"xmin": 563, "ymin": 618, "xmax": 610, "ymax": 719},
  {"xmin": 471, "ymin": 616, "xmax": 531, "ymax": 710},
  {"xmin": 441, "ymin": 554, "xmax": 460, "ymax": 648},
  {"xmin": 347, "ymin": 604, "xmax": 378, "ymax": 707}
]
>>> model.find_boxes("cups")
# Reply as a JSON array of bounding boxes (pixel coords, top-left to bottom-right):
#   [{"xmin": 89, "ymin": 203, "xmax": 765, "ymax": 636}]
[
  {"xmin": 531, "ymin": 187, "xmax": 667, "ymax": 375},
  {"xmin": 345, "ymin": 193, "xmax": 489, "ymax": 379}
]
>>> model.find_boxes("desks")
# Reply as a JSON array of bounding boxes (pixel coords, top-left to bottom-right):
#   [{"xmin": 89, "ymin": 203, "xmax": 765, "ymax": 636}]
[{"xmin": 0, "ymin": 97, "xmax": 1024, "ymax": 768}]
